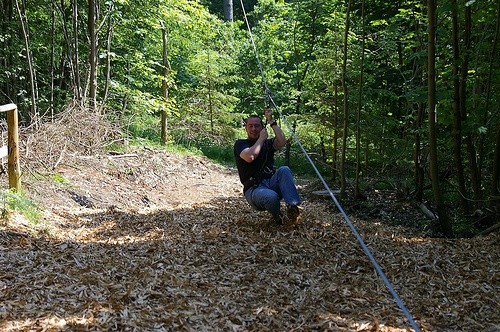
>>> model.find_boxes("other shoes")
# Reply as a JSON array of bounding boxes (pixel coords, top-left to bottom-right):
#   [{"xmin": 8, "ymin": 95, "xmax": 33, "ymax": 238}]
[
  {"xmin": 265, "ymin": 212, "xmax": 285, "ymax": 226},
  {"xmin": 286, "ymin": 205, "xmax": 299, "ymax": 223}
]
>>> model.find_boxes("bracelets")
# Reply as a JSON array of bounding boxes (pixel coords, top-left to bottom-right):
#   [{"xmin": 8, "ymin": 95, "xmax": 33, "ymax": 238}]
[{"xmin": 270, "ymin": 121, "xmax": 277, "ymax": 127}]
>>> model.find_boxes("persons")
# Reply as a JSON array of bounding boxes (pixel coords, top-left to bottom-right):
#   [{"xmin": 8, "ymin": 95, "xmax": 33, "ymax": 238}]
[{"xmin": 234, "ymin": 108, "xmax": 302, "ymax": 226}]
